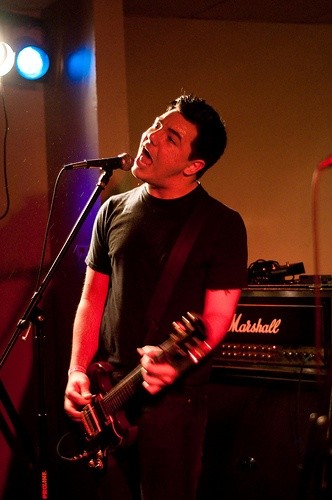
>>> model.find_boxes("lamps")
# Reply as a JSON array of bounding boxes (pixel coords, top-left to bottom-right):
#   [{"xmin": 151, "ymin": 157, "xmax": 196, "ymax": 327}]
[{"xmin": 16, "ymin": 39, "xmax": 51, "ymax": 83}]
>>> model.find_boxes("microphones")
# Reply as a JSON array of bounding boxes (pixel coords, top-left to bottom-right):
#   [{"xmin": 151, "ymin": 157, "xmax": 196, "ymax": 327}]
[{"xmin": 64, "ymin": 153, "xmax": 134, "ymax": 171}]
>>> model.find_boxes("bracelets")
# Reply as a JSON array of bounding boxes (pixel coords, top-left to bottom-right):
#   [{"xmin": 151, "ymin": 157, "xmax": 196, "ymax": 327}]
[{"xmin": 67, "ymin": 366, "xmax": 88, "ymax": 374}]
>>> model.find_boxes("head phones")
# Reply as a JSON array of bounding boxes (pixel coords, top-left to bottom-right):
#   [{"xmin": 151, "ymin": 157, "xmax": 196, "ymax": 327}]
[{"xmin": 247, "ymin": 261, "xmax": 305, "ymax": 285}]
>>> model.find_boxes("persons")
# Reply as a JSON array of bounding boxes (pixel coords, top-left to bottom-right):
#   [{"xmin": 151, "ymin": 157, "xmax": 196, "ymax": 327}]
[{"xmin": 63, "ymin": 92, "xmax": 249, "ymax": 500}]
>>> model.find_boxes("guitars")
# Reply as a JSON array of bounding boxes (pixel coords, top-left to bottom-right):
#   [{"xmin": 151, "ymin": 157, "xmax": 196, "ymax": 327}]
[{"xmin": 63, "ymin": 312, "xmax": 206, "ymax": 454}]
